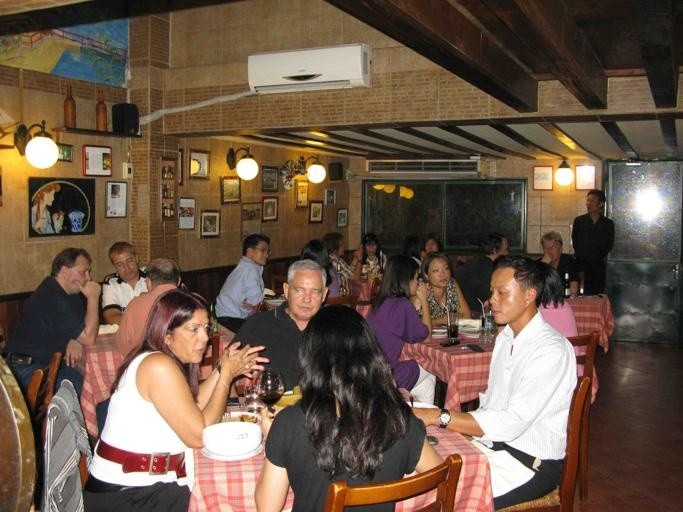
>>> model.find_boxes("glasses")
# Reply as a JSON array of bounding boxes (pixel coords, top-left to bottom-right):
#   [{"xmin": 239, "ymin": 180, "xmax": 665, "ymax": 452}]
[
  {"xmin": 289, "ymin": 281, "xmax": 325, "ymax": 297},
  {"xmin": 251, "ymin": 245, "xmax": 273, "ymax": 256}
]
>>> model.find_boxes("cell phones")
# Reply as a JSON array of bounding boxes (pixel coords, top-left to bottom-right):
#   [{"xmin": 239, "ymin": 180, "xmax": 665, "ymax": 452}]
[
  {"xmin": 440, "ymin": 339, "xmax": 461, "ymax": 347},
  {"xmin": 425, "ymin": 435, "xmax": 440, "ymax": 447},
  {"xmin": 227, "ymin": 397, "xmax": 240, "ymax": 406},
  {"xmin": 466, "ymin": 344, "xmax": 485, "ymax": 353}
]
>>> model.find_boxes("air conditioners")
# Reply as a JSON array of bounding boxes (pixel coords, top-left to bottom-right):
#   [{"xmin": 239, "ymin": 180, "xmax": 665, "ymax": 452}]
[{"xmin": 240, "ymin": 41, "xmax": 373, "ymax": 97}]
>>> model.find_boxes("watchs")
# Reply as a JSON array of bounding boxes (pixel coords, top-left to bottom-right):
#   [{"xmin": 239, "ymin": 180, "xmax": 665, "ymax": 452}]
[{"xmin": 437, "ymin": 408, "xmax": 449, "ymax": 428}]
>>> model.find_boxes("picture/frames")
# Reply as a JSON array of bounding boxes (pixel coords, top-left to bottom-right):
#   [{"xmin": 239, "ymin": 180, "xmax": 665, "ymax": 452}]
[
  {"xmin": 176, "ymin": 148, "xmax": 183, "ymax": 186},
  {"xmin": 240, "ymin": 166, "xmax": 350, "ymax": 244},
  {"xmin": 188, "ymin": 146, "xmax": 211, "ymax": 180},
  {"xmin": 573, "ymin": 164, "xmax": 597, "ymax": 191},
  {"xmin": 532, "ymin": 165, "xmax": 553, "ymax": 191},
  {"xmin": 102, "ymin": 180, "xmax": 127, "ymax": 218},
  {"xmin": 198, "ymin": 209, "xmax": 222, "ymax": 240},
  {"xmin": 79, "ymin": 143, "xmax": 113, "ymax": 179},
  {"xmin": 219, "ymin": 174, "xmax": 241, "ymax": 206},
  {"xmin": 178, "ymin": 197, "xmax": 196, "ymax": 232}
]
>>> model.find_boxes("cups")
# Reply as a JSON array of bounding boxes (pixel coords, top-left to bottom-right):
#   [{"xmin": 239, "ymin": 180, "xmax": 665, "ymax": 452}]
[
  {"xmin": 480, "ymin": 313, "xmax": 496, "ymax": 345},
  {"xmin": 445, "ymin": 313, "xmax": 458, "ymax": 342},
  {"xmin": 575, "ymin": 288, "xmax": 584, "ymax": 298}
]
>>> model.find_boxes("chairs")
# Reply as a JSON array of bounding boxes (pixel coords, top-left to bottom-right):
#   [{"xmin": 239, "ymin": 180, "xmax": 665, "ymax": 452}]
[
  {"xmin": 28, "ymin": 351, "xmax": 64, "ymax": 431},
  {"xmin": 44, "ymin": 275, "xmax": 616, "ymax": 512}
]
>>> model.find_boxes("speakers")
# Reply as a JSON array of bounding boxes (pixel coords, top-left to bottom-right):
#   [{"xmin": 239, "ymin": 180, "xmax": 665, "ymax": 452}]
[{"xmin": 328, "ymin": 162, "xmax": 343, "ymax": 180}]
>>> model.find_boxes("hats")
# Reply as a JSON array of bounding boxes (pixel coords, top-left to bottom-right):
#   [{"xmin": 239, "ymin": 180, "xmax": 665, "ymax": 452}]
[{"xmin": 361, "ymin": 233, "xmax": 380, "ymax": 245}]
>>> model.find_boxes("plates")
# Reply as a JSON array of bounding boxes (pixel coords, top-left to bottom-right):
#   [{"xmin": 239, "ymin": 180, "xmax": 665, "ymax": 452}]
[
  {"xmin": 431, "ymin": 319, "xmax": 497, "ymax": 334},
  {"xmin": 201, "ymin": 421, "xmax": 264, "ymax": 462},
  {"xmin": 220, "ymin": 410, "xmax": 260, "ymax": 423}
]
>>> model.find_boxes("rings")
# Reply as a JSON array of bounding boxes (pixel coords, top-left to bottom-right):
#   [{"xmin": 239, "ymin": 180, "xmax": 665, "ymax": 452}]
[{"xmin": 244, "ymin": 364, "xmax": 251, "ymax": 371}]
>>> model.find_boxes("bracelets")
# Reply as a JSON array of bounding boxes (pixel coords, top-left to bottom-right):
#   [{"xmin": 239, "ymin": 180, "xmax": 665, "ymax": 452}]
[{"xmin": 215, "ymin": 360, "xmax": 221, "ymax": 374}]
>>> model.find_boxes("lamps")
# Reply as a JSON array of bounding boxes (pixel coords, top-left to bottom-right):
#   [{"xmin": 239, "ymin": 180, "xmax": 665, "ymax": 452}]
[
  {"xmin": 292, "ymin": 152, "xmax": 326, "ymax": 185},
  {"xmin": 0, "ymin": 118, "xmax": 59, "ymax": 172},
  {"xmin": 553, "ymin": 157, "xmax": 574, "ymax": 186},
  {"xmin": 225, "ymin": 144, "xmax": 260, "ymax": 182}
]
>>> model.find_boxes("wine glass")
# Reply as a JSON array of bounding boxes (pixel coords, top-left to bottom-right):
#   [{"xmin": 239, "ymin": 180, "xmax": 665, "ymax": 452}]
[{"xmin": 254, "ymin": 370, "xmax": 285, "ymax": 420}]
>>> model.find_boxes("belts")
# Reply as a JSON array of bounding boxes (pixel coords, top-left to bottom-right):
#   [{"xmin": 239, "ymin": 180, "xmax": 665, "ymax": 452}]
[{"xmin": 94, "ymin": 437, "xmax": 184, "ymax": 474}]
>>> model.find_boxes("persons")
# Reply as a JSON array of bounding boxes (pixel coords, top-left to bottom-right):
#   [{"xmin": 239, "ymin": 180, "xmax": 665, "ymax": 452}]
[
  {"xmin": 570, "ymin": 189, "xmax": 614, "ymax": 294},
  {"xmin": 82, "ymin": 288, "xmax": 270, "ymax": 512},
  {"xmin": 4, "ymin": 247, "xmax": 101, "ymax": 397},
  {"xmin": 255, "ymin": 303, "xmax": 445, "ymax": 510},
  {"xmin": 31, "ymin": 184, "xmax": 54, "ymax": 234},
  {"xmin": 537, "ymin": 232, "xmax": 580, "ymax": 297},
  {"xmin": 112, "ymin": 257, "xmax": 209, "ymax": 357},
  {"xmin": 101, "ymin": 241, "xmax": 147, "ymax": 324},
  {"xmin": 408, "ymin": 252, "xmax": 577, "ymax": 510}
]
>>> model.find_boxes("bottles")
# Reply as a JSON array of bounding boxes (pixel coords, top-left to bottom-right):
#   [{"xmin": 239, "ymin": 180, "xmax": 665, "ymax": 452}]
[
  {"xmin": 94, "ymin": 90, "xmax": 107, "ymax": 132},
  {"xmin": 63, "ymin": 84, "xmax": 76, "ymax": 130},
  {"xmin": 563, "ymin": 271, "xmax": 570, "ymax": 299}
]
